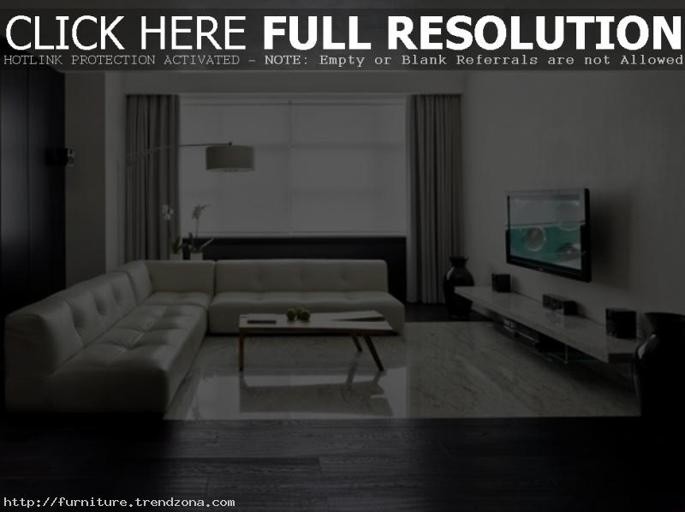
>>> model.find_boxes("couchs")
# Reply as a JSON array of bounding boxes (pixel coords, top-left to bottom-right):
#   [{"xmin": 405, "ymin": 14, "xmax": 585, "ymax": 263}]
[{"xmin": 1, "ymin": 258, "xmax": 407, "ymax": 409}]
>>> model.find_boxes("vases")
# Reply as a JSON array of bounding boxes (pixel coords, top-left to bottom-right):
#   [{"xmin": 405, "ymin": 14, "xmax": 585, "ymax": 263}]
[
  {"xmin": 443, "ymin": 255, "xmax": 473, "ymax": 316},
  {"xmin": 633, "ymin": 310, "xmax": 685, "ymax": 418},
  {"xmin": 169, "ymin": 253, "xmax": 204, "ymax": 260}
]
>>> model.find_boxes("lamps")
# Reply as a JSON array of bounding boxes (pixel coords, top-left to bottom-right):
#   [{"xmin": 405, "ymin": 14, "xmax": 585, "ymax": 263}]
[
  {"xmin": 127, "ymin": 142, "xmax": 255, "ymax": 172},
  {"xmin": 44, "ymin": 147, "xmax": 91, "ymax": 189}
]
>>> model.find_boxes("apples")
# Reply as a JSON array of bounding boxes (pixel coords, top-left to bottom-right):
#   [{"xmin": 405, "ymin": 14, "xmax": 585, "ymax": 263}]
[{"xmin": 286, "ymin": 306, "xmax": 310, "ymax": 321}]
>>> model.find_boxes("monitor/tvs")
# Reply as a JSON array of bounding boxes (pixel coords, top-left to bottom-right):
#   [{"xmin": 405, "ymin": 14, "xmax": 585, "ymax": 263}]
[{"xmin": 504, "ymin": 190, "xmax": 592, "ymax": 282}]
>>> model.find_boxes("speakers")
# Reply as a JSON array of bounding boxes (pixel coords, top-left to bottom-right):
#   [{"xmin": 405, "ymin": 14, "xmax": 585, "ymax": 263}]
[
  {"xmin": 605, "ymin": 307, "xmax": 638, "ymax": 340},
  {"xmin": 543, "ymin": 293, "xmax": 578, "ymax": 315},
  {"xmin": 491, "ymin": 272, "xmax": 511, "ymax": 292}
]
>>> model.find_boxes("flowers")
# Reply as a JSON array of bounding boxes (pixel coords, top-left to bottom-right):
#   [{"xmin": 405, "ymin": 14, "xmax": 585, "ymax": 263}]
[{"xmin": 159, "ymin": 202, "xmax": 216, "ymax": 253}]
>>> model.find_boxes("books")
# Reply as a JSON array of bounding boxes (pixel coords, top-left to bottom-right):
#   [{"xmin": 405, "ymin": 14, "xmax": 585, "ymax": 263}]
[{"xmin": 247, "ymin": 313, "xmax": 279, "ymax": 324}]
